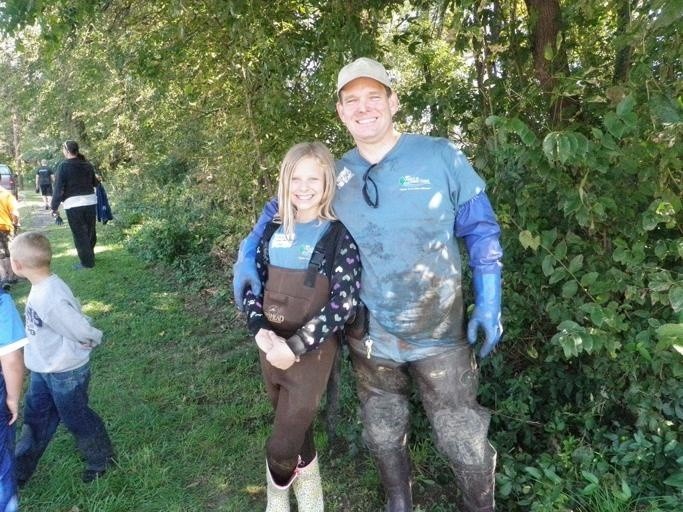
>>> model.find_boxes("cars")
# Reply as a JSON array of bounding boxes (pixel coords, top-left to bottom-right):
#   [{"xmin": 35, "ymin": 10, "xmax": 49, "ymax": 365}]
[{"xmin": 0, "ymin": 164, "xmax": 19, "ymax": 202}]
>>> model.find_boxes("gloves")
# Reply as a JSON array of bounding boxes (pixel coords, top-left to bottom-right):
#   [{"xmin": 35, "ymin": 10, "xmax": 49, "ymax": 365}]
[
  {"xmin": 233, "ymin": 197, "xmax": 281, "ymax": 311},
  {"xmin": 455, "ymin": 191, "xmax": 503, "ymax": 358},
  {"xmin": 55, "ymin": 214, "xmax": 63, "ymax": 226}
]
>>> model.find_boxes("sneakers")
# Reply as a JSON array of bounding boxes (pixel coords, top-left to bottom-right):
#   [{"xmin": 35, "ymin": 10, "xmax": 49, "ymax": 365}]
[{"xmin": 83, "ymin": 470, "xmax": 104, "ymax": 483}]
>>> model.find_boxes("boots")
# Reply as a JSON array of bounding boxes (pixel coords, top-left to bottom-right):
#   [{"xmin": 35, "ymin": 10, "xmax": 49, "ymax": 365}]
[
  {"xmin": 371, "ymin": 445, "xmax": 414, "ymax": 512},
  {"xmin": 451, "ymin": 440, "xmax": 497, "ymax": 512},
  {"xmin": 265, "ymin": 450, "xmax": 324, "ymax": 512}
]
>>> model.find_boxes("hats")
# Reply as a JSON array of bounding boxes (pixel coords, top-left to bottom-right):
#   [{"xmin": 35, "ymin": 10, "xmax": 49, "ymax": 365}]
[{"xmin": 337, "ymin": 57, "xmax": 392, "ymax": 96}]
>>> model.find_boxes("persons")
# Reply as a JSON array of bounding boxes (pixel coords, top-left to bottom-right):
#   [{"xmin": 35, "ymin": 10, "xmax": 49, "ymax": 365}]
[
  {"xmin": 35, "ymin": 158, "xmax": 55, "ymax": 211},
  {"xmin": 0, "ymin": 173, "xmax": 24, "ymax": 290},
  {"xmin": 232, "ymin": 56, "xmax": 504, "ymax": 510},
  {"xmin": 241, "ymin": 139, "xmax": 362, "ymax": 512},
  {"xmin": 0, "ymin": 289, "xmax": 31, "ymax": 511},
  {"xmin": 49, "ymin": 139, "xmax": 97, "ymax": 269},
  {"xmin": 10, "ymin": 232, "xmax": 116, "ymax": 487}
]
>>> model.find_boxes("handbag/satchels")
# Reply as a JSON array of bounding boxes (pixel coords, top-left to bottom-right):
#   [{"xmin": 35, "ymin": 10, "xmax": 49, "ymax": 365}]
[{"xmin": 344, "ymin": 300, "xmax": 369, "ymax": 340}]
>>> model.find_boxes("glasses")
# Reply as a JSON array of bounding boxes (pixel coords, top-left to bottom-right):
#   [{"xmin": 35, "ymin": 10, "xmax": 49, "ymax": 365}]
[{"xmin": 362, "ymin": 163, "xmax": 378, "ymax": 208}]
